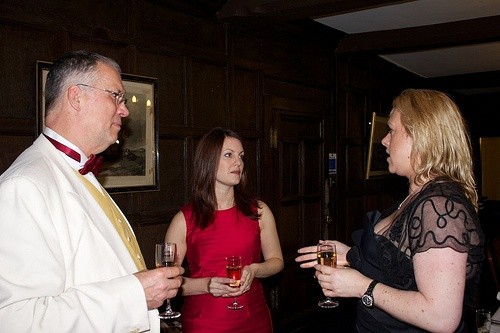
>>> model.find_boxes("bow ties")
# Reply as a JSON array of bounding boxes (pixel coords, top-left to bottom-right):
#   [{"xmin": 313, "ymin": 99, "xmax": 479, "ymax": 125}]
[{"xmin": 42, "ymin": 133, "xmax": 103, "ymax": 177}]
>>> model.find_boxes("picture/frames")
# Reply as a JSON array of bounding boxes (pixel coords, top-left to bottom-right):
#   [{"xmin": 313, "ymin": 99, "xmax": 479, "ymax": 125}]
[
  {"xmin": 35, "ymin": 61, "xmax": 160, "ymax": 194},
  {"xmin": 365, "ymin": 112, "xmax": 390, "ymax": 179}
]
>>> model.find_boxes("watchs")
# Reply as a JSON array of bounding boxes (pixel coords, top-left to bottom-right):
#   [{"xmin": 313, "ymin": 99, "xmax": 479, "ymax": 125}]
[{"xmin": 362, "ymin": 279, "xmax": 380, "ymax": 308}]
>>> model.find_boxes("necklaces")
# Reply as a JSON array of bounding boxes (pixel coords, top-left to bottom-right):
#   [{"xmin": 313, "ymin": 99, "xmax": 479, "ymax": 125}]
[{"xmin": 397, "ymin": 193, "xmax": 412, "ymax": 210}]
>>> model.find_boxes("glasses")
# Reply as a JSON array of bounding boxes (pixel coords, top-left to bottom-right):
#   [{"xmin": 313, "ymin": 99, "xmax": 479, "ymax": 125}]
[{"xmin": 76, "ymin": 84, "xmax": 127, "ymax": 106}]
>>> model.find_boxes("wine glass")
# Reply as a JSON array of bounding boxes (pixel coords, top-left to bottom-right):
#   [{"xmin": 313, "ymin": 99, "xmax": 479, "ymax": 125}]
[
  {"xmin": 154, "ymin": 243, "xmax": 181, "ymax": 319},
  {"xmin": 318, "ymin": 242, "xmax": 339, "ymax": 308},
  {"xmin": 225, "ymin": 256, "xmax": 244, "ymax": 309}
]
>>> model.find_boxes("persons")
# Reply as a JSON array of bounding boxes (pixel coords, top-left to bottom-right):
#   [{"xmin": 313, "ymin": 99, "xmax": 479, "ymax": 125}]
[
  {"xmin": 164, "ymin": 125, "xmax": 285, "ymax": 333},
  {"xmin": 0, "ymin": 50, "xmax": 186, "ymax": 332},
  {"xmin": 295, "ymin": 88, "xmax": 485, "ymax": 333}
]
